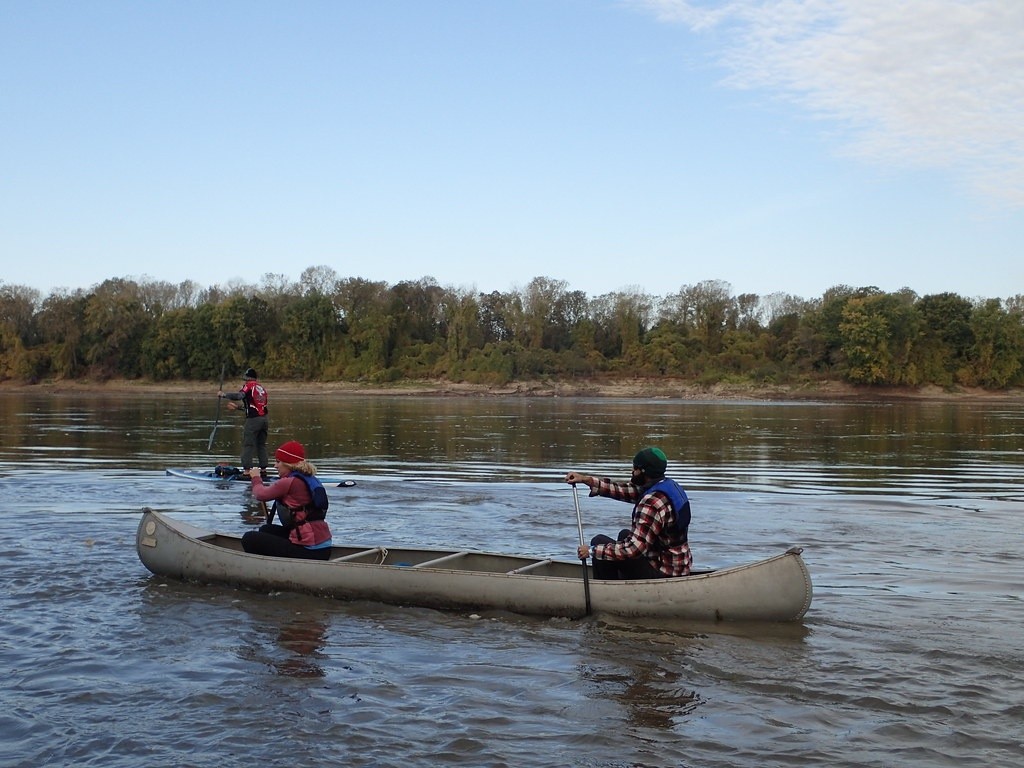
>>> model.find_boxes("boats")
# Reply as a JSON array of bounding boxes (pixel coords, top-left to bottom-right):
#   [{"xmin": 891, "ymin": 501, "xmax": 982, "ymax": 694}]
[
  {"xmin": 167, "ymin": 467, "xmax": 356, "ymax": 487},
  {"xmin": 136, "ymin": 507, "xmax": 814, "ymax": 642}
]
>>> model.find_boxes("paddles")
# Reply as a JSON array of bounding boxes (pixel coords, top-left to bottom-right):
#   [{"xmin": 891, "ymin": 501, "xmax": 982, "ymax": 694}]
[
  {"xmin": 208, "ymin": 360, "xmax": 226, "ymax": 451},
  {"xmin": 566, "ymin": 475, "xmax": 592, "ymax": 622},
  {"xmin": 250, "ymin": 467, "xmax": 269, "ymax": 521}
]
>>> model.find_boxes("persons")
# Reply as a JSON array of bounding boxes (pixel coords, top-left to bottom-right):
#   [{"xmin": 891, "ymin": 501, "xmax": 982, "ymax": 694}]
[
  {"xmin": 567, "ymin": 447, "xmax": 692, "ymax": 579},
  {"xmin": 218, "ymin": 368, "xmax": 269, "ymax": 477},
  {"xmin": 241, "ymin": 441, "xmax": 332, "ymax": 560}
]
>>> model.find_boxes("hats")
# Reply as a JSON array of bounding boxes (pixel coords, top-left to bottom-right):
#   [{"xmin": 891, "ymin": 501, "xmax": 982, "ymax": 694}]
[
  {"xmin": 274, "ymin": 441, "xmax": 304, "ymax": 464},
  {"xmin": 633, "ymin": 447, "xmax": 668, "ymax": 477},
  {"xmin": 243, "ymin": 368, "xmax": 257, "ymax": 377}
]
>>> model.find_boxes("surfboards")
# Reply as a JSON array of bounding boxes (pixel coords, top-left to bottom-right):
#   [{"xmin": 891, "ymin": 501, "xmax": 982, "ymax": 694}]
[{"xmin": 167, "ymin": 468, "xmax": 357, "ymax": 488}]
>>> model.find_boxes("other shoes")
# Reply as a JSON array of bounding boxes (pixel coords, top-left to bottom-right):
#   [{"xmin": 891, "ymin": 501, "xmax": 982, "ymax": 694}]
[
  {"xmin": 234, "ymin": 474, "xmax": 252, "ymax": 481},
  {"xmin": 260, "ymin": 471, "xmax": 268, "ymax": 479}
]
